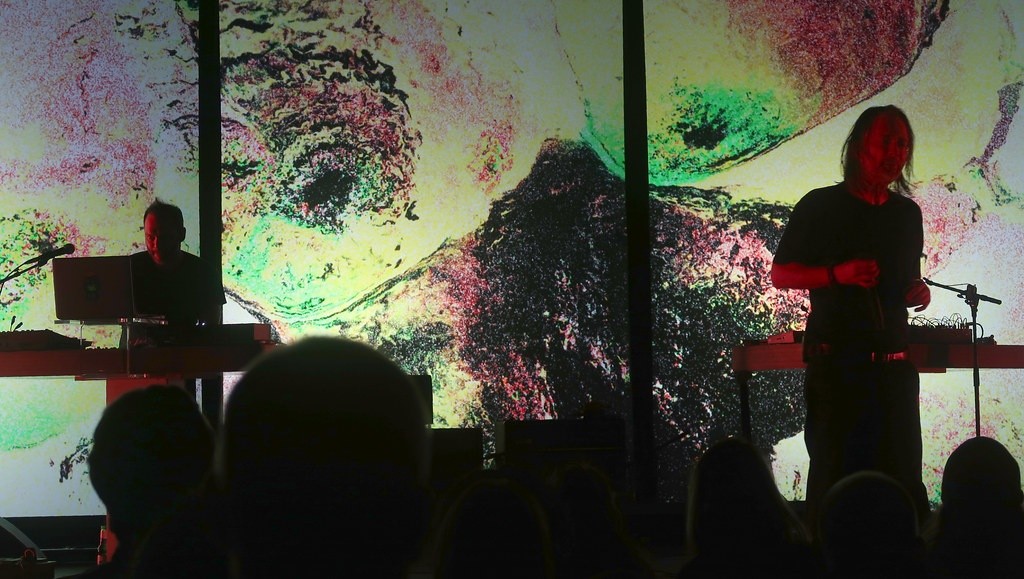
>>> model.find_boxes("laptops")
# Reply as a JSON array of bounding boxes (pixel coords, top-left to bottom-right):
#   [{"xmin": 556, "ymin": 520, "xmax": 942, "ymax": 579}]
[{"xmin": 52, "ymin": 256, "xmax": 165, "ymax": 321}]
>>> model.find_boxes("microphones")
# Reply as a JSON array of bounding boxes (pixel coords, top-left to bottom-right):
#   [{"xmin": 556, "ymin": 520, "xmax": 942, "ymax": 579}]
[{"xmin": 25, "ymin": 244, "xmax": 75, "ymax": 264}]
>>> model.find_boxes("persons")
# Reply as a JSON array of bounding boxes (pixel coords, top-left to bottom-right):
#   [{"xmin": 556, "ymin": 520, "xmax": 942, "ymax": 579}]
[
  {"xmin": 58, "ymin": 340, "xmax": 1024, "ymax": 579},
  {"xmin": 118, "ymin": 198, "xmax": 226, "ymax": 349},
  {"xmin": 772, "ymin": 106, "xmax": 930, "ymax": 543}
]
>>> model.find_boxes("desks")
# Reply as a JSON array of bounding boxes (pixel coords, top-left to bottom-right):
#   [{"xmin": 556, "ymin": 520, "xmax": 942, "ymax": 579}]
[
  {"xmin": 0, "ymin": 343, "xmax": 286, "ymax": 564},
  {"xmin": 733, "ymin": 344, "xmax": 1024, "ymax": 445}
]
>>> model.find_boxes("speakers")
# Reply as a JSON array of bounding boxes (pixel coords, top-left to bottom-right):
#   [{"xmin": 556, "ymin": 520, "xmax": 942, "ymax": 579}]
[
  {"xmin": 494, "ymin": 416, "xmax": 627, "ymax": 505},
  {"xmin": 426, "ymin": 428, "xmax": 484, "ymax": 476}
]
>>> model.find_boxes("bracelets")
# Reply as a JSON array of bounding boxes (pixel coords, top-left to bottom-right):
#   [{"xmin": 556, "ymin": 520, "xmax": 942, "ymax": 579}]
[{"xmin": 829, "ymin": 264, "xmax": 835, "ymax": 283}]
